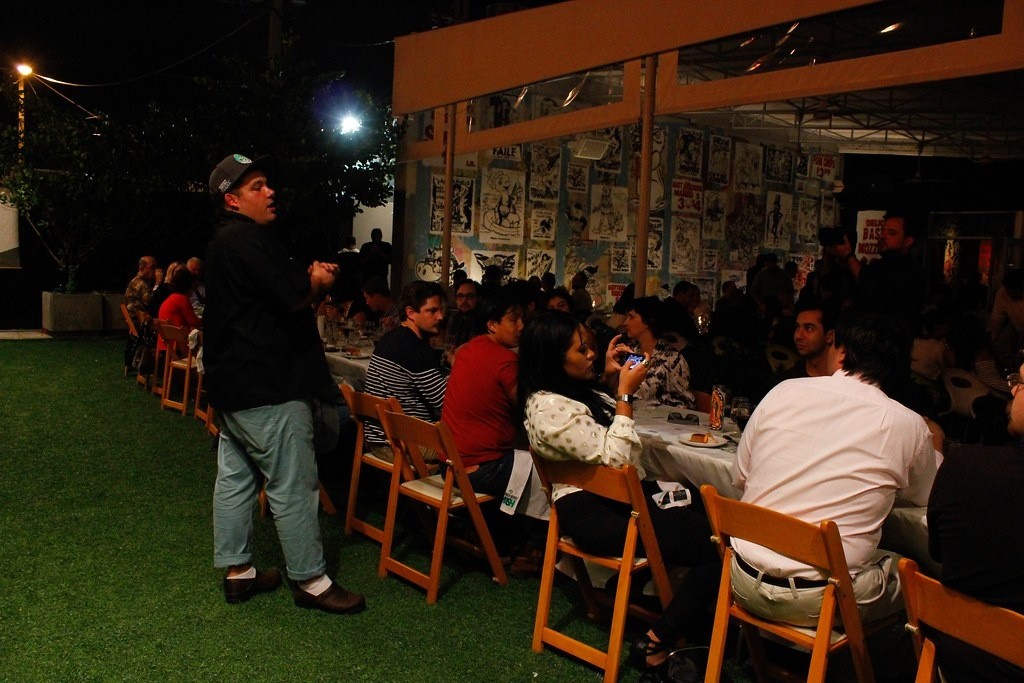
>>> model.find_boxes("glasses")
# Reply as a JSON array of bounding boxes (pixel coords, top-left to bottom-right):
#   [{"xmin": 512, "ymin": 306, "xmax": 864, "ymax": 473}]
[
  {"xmin": 1006, "ymin": 373, "xmax": 1024, "ymax": 387},
  {"xmin": 457, "ymin": 293, "xmax": 478, "ymax": 299}
]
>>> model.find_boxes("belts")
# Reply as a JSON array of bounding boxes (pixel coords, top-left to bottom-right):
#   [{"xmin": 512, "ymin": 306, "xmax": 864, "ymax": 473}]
[{"xmin": 733, "ymin": 548, "xmax": 829, "ymax": 588}]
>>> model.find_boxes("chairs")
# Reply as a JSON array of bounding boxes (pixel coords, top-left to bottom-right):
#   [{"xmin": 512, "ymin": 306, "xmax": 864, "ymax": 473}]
[
  {"xmin": 378, "ymin": 405, "xmax": 508, "ymax": 602},
  {"xmin": 701, "ymin": 483, "xmax": 904, "ymax": 683},
  {"xmin": 897, "ymin": 558, "xmax": 1024, "ymax": 683},
  {"xmin": 338, "ymin": 383, "xmax": 441, "ymax": 544},
  {"xmin": 120, "ymin": 302, "xmax": 217, "ymax": 438},
  {"xmin": 692, "ymin": 391, "xmax": 710, "ymax": 410},
  {"xmin": 527, "ymin": 443, "xmax": 676, "ymax": 683}
]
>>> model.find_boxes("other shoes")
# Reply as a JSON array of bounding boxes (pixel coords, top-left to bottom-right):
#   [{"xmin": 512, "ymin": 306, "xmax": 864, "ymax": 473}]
[{"xmin": 510, "ymin": 554, "xmax": 546, "ymax": 580}]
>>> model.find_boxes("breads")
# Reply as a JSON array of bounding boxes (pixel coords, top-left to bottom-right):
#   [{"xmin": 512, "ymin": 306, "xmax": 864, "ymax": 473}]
[{"xmin": 691, "ymin": 433, "xmax": 709, "ymax": 443}]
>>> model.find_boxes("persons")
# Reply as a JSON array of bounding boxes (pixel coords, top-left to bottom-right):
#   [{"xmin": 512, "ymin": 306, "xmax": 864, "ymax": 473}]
[
  {"xmin": 124, "ymin": 255, "xmax": 206, "ymax": 405},
  {"xmin": 209, "ymin": 153, "xmax": 366, "ymax": 613},
  {"xmin": 927, "ymin": 364, "xmax": 1024, "ymax": 683},
  {"xmin": 312, "ymin": 206, "xmax": 1024, "ymax": 683}
]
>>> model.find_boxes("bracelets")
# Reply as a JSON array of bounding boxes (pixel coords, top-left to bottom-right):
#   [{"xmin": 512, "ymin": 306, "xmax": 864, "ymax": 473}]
[{"xmin": 844, "ymin": 252, "xmax": 856, "ymax": 262}]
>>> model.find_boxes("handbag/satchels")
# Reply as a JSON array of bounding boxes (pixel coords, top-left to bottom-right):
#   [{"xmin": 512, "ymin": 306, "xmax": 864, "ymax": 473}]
[{"xmin": 125, "ymin": 345, "xmax": 156, "ymax": 373}]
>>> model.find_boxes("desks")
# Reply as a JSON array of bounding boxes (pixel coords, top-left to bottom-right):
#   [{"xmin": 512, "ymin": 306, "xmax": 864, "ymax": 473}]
[
  {"xmin": 317, "ymin": 339, "xmax": 376, "ymax": 418},
  {"xmin": 627, "ymin": 396, "xmax": 945, "ymax": 587}
]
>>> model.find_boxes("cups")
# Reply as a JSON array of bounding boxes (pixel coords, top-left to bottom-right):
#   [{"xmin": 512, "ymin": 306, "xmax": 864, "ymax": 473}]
[
  {"xmin": 731, "ymin": 396, "xmax": 749, "ymax": 425},
  {"xmin": 737, "ymin": 403, "xmax": 756, "ymax": 431},
  {"xmin": 322, "ymin": 305, "xmax": 375, "ymax": 353}
]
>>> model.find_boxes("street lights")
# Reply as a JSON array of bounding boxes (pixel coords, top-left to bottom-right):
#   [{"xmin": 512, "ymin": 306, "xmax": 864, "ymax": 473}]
[{"xmin": 15, "ymin": 62, "xmax": 34, "ymax": 181}]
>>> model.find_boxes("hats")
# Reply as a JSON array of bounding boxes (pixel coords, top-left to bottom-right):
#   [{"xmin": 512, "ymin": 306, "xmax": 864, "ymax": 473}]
[
  {"xmin": 633, "ymin": 296, "xmax": 659, "ymax": 323},
  {"xmin": 209, "ymin": 154, "xmax": 266, "ymax": 197}
]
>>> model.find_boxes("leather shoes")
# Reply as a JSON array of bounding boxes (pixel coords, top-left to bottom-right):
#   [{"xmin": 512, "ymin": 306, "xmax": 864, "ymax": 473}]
[
  {"xmin": 295, "ymin": 579, "xmax": 366, "ymax": 615},
  {"xmin": 224, "ymin": 567, "xmax": 280, "ymax": 603}
]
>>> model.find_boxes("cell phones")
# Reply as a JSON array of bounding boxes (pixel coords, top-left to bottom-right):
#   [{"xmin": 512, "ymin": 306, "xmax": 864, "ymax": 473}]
[{"xmin": 625, "ymin": 353, "xmax": 645, "ymax": 369}]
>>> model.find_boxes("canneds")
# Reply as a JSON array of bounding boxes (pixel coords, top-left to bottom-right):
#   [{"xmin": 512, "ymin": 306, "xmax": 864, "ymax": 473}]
[{"xmin": 709, "ymin": 385, "xmax": 727, "ymax": 430}]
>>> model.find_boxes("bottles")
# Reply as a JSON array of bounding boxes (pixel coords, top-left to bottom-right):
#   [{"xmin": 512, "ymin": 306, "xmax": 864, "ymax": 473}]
[
  {"xmin": 323, "ymin": 295, "xmax": 332, "ymax": 331},
  {"xmin": 709, "ymin": 385, "xmax": 727, "ymax": 432}
]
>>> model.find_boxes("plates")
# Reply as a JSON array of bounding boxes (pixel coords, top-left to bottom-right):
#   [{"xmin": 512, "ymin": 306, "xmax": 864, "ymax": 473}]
[
  {"xmin": 344, "ymin": 352, "xmax": 369, "ymax": 359},
  {"xmin": 677, "ymin": 433, "xmax": 728, "ymax": 447}
]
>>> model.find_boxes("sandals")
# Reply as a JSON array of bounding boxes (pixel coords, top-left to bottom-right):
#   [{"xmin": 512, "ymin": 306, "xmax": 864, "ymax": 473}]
[{"xmin": 627, "ymin": 635, "xmax": 676, "ymax": 683}]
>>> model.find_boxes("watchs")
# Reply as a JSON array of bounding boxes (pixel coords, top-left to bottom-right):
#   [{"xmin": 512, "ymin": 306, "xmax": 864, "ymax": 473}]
[{"xmin": 616, "ymin": 393, "xmax": 633, "ymax": 405}]
